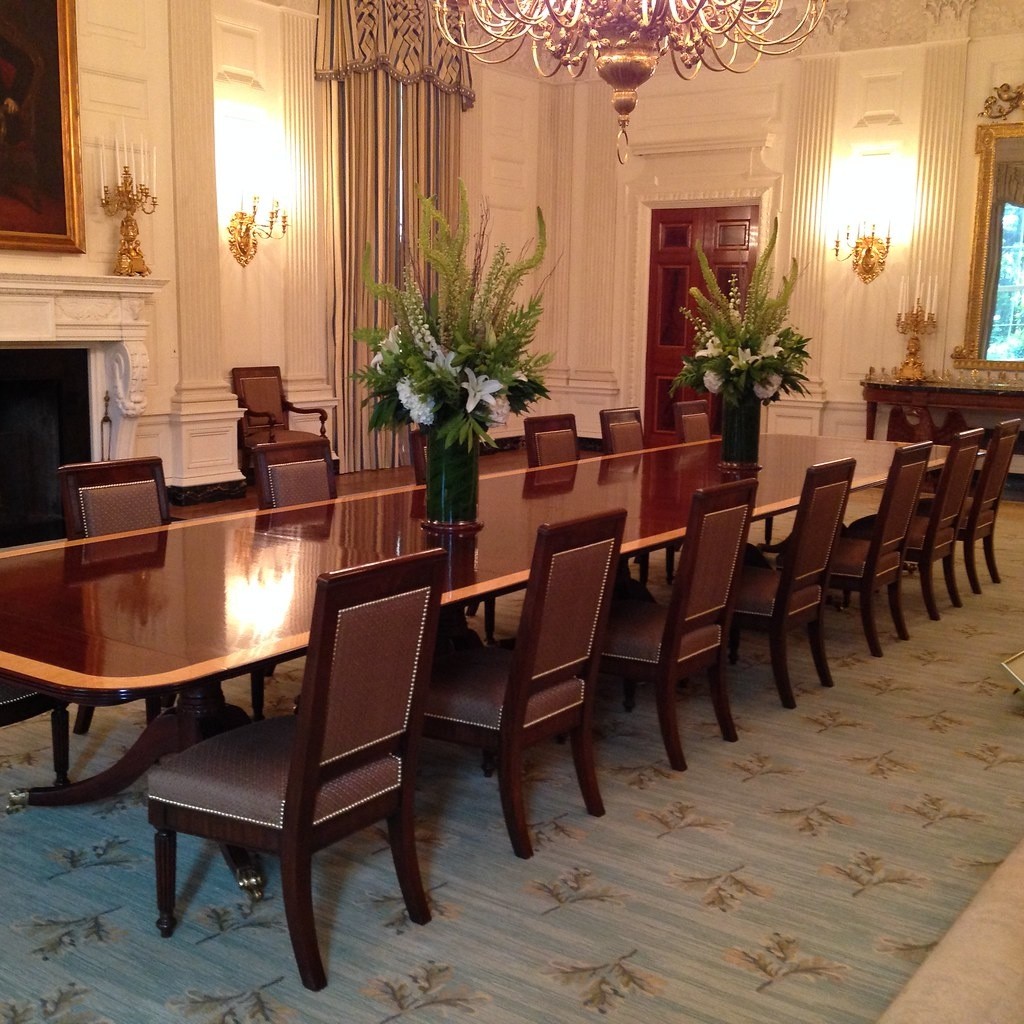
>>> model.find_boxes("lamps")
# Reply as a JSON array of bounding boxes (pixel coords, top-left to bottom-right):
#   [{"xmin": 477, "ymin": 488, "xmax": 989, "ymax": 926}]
[
  {"xmin": 227, "ymin": 188, "xmax": 287, "ymax": 266},
  {"xmin": 426, "ymin": 0, "xmax": 857, "ymax": 163}
]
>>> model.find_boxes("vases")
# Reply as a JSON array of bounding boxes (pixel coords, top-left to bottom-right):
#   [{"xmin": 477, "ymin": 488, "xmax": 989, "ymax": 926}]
[
  {"xmin": 419, "ymin": 416, "xmax": 486, "ymax": 538},
  {"xmin": 720, "ymin": 391, "xmax": 763, "ymax": 472}
]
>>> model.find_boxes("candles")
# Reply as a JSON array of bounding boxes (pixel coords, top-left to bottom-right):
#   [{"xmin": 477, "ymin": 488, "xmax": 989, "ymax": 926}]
[
  {"xmin": 835, "ymin": 213, "xmax": 891, "ymax": 283},
  {"xmin": 97, "ymin": 115, "xmax": 158, "ymax": 201},
  {"xmin": 896, "ymin": 260, "xmax": 939, "ymax": 319}
]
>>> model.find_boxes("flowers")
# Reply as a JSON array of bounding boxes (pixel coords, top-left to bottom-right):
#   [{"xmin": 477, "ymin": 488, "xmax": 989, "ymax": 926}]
[
  {"xmin": 359, "ymin": 187, "xmax": 557, "ymax": 522},
  {"xmin": 666, "ymin": 217, "xmax": 814, "ymax": 460}
]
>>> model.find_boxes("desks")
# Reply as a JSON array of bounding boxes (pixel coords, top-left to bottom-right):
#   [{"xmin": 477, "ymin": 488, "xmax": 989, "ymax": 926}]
[
  {"xmin": 859, "ymin": 379, "xmax": 1024, "ymax": 439},
  {"xmin": 0, "ymin": 433, "xmax": 945, "ymax": 903}
]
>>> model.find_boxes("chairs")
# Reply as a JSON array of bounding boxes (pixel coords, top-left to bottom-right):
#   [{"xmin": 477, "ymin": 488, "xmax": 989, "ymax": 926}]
[{"xmin": 52, "ymin": 363, "xmax": 1022, "ymax": 995}]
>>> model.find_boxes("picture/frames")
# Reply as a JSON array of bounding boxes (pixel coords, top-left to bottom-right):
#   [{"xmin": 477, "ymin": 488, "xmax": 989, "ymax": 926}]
[{"xmin": 0, "ymin": 1, "xmax": 87, "ymax": 254}]
